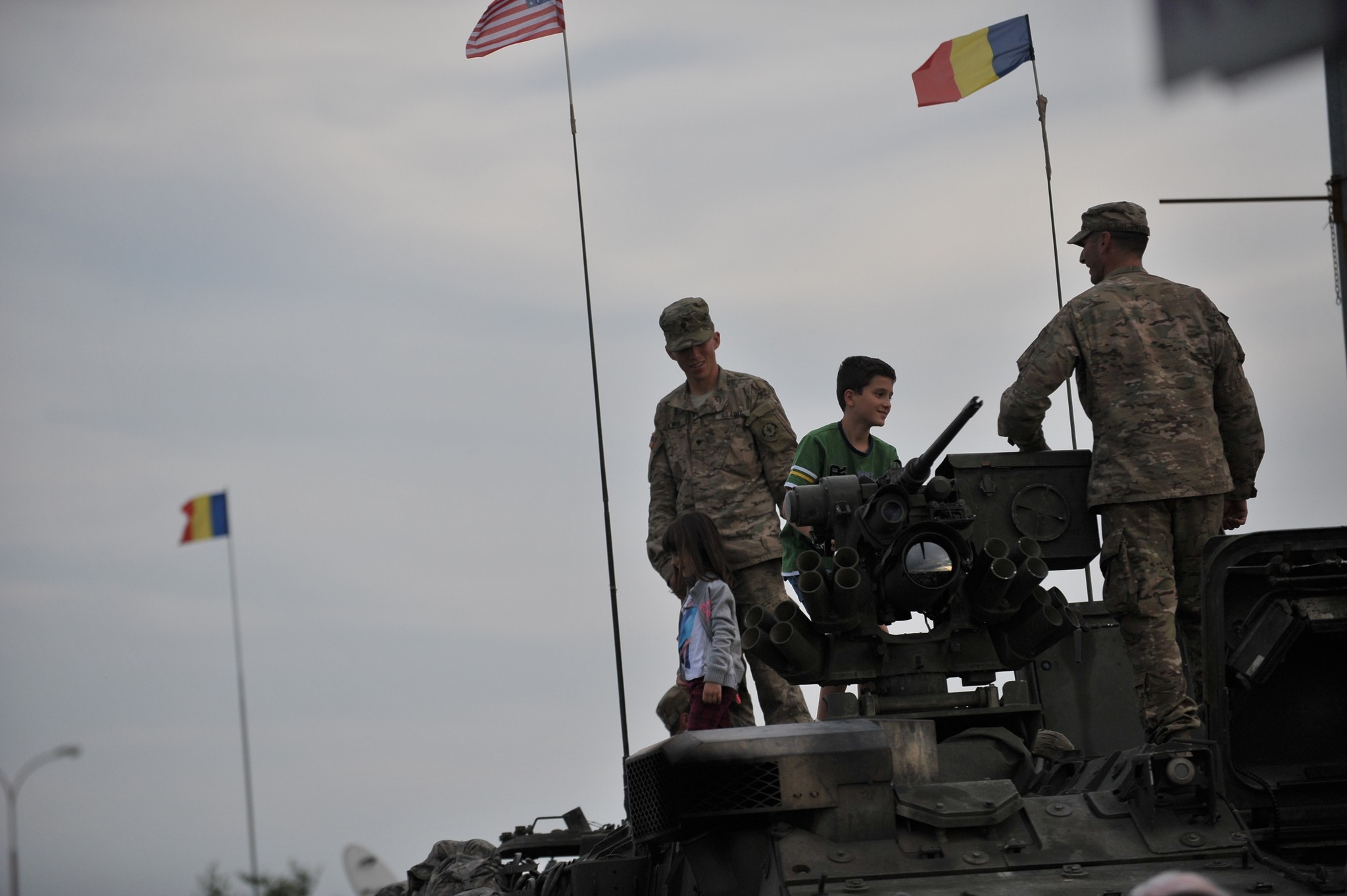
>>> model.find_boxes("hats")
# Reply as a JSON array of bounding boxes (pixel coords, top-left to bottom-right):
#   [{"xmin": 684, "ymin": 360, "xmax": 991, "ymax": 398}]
[
  {"xmin": 1066, "ymin": 201, "xmax": 1150, "ymax": 244},
  {"xmin": 659, "ymin": 296, "xmax": 715, "ymax": 352}
]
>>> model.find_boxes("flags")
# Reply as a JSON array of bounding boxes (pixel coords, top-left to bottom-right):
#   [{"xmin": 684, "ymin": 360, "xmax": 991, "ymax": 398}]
[
  {"xmin": 182, "ymin": 493, "xmax": 227, "ymax": 542},
  {"xmin": 912, "ymin": 15, "xmax": 1036, "ymax": 107},
  {"xmin": 466, "ymin": 0, "xmax": 565, "ymax": 59}
]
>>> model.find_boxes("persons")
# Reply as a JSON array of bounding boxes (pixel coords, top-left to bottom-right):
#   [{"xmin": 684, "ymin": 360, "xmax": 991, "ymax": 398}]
[
  {"xmin": 646, "ymin": 297, "xmax": 806, "ymax": 726},
  {"xmin": 661, "ymin": 513, "xmax": 745, "ymax": 731},
  {"xmin": 656, "ymin": 685, "xmax": 691, "ymax": 735},
  {"xmin": 998, "ymin": 201, "xmax": 1265, "ymax": 755},
  {"xmin": 777, "ymin": 356, "xmax": 902, "ymax": 718}
]
